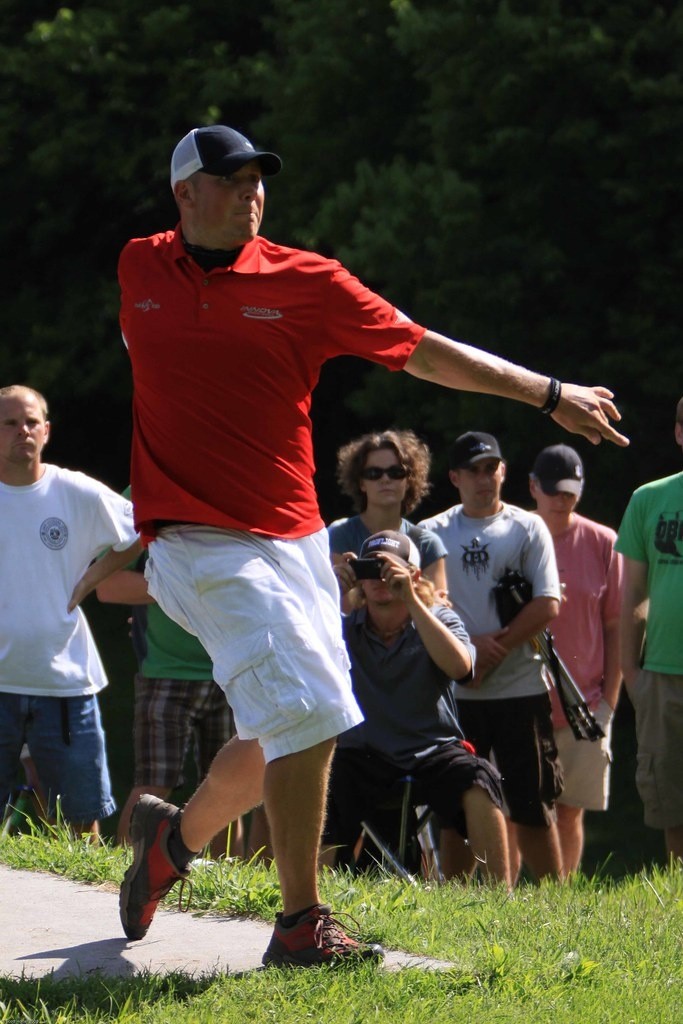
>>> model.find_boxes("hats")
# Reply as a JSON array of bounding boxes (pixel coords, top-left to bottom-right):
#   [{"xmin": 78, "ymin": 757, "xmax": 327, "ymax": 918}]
[
  {"xmin": 360, "ymin": 530, "xmax": 421, "ymax": 570},
  {"xmin": 532, "ymin": 443, "xmax": 583, "ymax": 495},
  {"xmin": 450, "ymin": 432, "xmax": 503, "ymax": 471},
  {"xmin": 170, "ymin": 125, "xmax": 282, "ymax": 195}
]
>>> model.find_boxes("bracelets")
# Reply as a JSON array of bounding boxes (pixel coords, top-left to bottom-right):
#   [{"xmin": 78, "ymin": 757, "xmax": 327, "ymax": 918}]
[
  {"xmin": 81, "ymin": 576, "xmax": 98, "ymax": 591},
  {"xmin": 538, "ymin": 377, "xmax": 563, "ymax": 418}
]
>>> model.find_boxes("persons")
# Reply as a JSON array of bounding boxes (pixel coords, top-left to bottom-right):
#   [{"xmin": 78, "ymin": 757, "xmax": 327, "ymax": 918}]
[
  {"xmin": 0, "ymin": 384, "xmax": 245, "ymax": 866},
  {"xmin": 119, "ymin": 124, "xmax": 631, "ymax": 971},
  {"xmin": 248, "ymin": 399, "xmax": 683, "ymax": 897}
]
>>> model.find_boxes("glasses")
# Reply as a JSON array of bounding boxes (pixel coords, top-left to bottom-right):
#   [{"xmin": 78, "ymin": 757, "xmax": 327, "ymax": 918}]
[{"xmin": 357, "ymin": 465, "xmax": 409, "ymax": 481}]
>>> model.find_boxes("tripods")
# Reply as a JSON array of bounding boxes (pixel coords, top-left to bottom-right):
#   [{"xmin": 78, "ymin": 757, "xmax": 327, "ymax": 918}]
[{"xmin": 494, "ymin": 569, "xmax": 606, "ymax": 741}]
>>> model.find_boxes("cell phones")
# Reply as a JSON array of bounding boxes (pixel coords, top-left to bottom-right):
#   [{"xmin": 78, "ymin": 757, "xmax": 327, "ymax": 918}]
[{"xmin": 348, "ymin": 558, "xmax": 386, "ymax": 580}]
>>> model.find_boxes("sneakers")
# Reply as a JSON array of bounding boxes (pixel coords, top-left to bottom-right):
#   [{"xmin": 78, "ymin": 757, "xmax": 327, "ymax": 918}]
[
  {"xmin": 261, "ymin": 904, "xmax": 383, "ymax": 966},
  {"xmin": 119, "ymin": 794, "xmax": 192, "ymax": 940}
]
